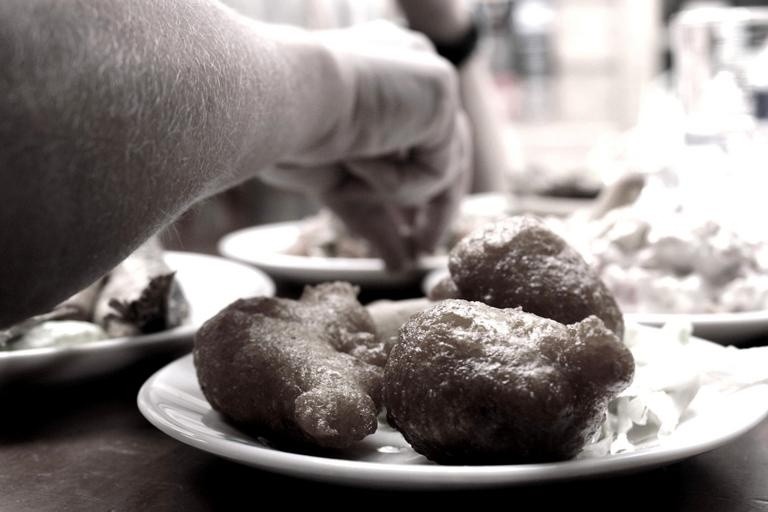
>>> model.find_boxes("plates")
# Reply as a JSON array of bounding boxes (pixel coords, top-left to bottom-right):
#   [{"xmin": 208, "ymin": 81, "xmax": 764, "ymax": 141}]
[
  {"xmin": 214, "ymin": 213, "xmax": 493, "ymax": 283},
  {"xmin": 1, "ymin": 245, "xmax": 276, "ymax": 384},
  {"xmin": 569, "ymin": 216, "xmax": 768, "ymax": 342},
  {"xmin": 135, "ymin": 291, "xmax": 768, "ymax": 487}
]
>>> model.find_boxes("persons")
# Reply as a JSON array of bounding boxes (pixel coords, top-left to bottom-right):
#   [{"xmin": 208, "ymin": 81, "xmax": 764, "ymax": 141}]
[
  {"xmin": 155, "ymin": 0, "xmax": 510, "ymax": 257},
  {"xmin": 0, "ymin": 0, "xmax": 460, "ymax": 329}
]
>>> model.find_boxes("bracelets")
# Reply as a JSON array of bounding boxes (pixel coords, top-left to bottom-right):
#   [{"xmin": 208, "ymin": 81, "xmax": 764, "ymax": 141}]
[{"xmin": 434, "ymin": 11, "xmax": 480, "ymax": 66}]
